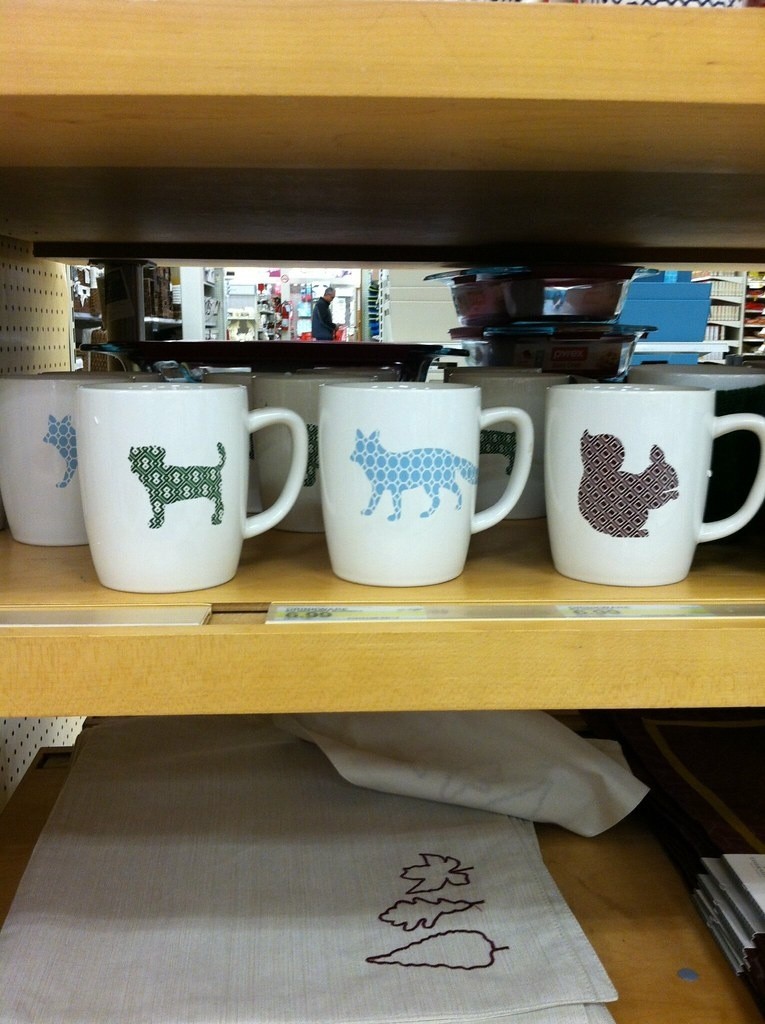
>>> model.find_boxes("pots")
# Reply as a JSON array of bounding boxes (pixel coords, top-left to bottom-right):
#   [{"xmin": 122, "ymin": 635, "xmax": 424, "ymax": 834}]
[
  {"xmin": 79, "ymin": 342, "xmax": 471, "ymax": 391},
  {"xmin": 422, "ymin": 261, "xmax": 660, "ymax": 376}
]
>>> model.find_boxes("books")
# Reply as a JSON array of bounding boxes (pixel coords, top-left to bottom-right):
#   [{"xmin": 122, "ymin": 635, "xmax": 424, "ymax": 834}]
[{"xmin": 693, "ymin": 270, "xmax": 743, "ymax": 341}]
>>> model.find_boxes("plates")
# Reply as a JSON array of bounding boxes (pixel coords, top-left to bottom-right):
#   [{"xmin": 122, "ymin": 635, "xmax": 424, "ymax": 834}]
[{"xmin": 630, "ymin": 366, "xmax": 765, "ymax": 393}]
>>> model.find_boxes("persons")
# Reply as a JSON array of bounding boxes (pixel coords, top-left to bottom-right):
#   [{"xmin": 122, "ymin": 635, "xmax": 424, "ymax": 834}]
[{"xmin": 312, "ymin": 287, "xmax": 339, "ymax": 341}]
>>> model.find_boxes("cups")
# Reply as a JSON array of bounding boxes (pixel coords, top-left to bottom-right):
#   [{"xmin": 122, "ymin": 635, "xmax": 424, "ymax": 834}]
[{"xmin": 2, "ymin": 364, "xmax": 765, "ymax": 589}]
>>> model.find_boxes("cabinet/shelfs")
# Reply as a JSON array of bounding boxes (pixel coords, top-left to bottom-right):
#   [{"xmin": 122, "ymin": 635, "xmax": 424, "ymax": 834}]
[
  {"xmin": 742, "ymin": 294, "xmax": 765, "ymax": 357},
  {"xmin": 179, "ymin": 266, "xmax": 227, "ymax": 340},
  {"xmin": 0, "ymin": 0, "xmax": 765, "ymax": 1024},
  {"xmin": 691, "ymin": 269, "xmax": 747, "ymax": 365}
]
